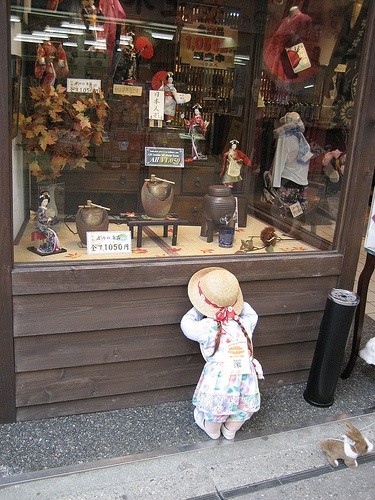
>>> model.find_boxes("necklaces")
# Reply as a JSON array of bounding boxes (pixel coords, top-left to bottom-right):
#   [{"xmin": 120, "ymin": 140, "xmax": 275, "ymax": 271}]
[{"xmin": 352, "ymin": 4, "xmax": 356, "ymax": 26}]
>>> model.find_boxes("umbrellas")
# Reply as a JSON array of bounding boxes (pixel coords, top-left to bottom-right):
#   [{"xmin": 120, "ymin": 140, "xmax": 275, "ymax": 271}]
[
  {"xmin": 152, "ymin": 70, "xmax": 170, "ymax": 90},
  {"xmin": 136, "ymin": 36, "xmax": 154, "ymax": 58}
]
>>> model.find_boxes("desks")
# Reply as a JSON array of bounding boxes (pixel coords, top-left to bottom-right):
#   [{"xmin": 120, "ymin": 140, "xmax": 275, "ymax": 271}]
[
  {"xmin": 120, "ymin": 212, "xmax": 190, "ymax": 248},
  {"xmin": 273, "ymin": 119, "xmax": 349, "ymax": 183}
]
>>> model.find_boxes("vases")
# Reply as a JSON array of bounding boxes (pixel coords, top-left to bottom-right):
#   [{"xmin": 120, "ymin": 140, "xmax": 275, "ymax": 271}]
[{"xmin": 37, "ymin": 180, "xmax": 66, "ymax": 233}]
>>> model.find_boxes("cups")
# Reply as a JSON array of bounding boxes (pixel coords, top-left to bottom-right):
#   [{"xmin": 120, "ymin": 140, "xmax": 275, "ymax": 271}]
[{"xmin": 219, "ymin": 227, "xmax": 234, "ymax": 248}]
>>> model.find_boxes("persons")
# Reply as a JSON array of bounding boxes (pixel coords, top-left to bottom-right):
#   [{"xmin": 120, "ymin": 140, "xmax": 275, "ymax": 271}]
[
  {"xmin": 311, "ymin": 0, "xmax": 371, "ymax": 226},
  {"xmin": 181, "ymin": 267, "xmax": 265, "ymax": 440},
  {"xmin": 17, "ymin": 0, "xmax": 58, "ymax": 32},
  {"xmin": 269, "ymin": 6, "xmax": 320, "ymax": 81},
  {"xmin": 122, "ymin": 32, "xmax": 144, "ymax": 86},
  {"xmin": 33, "ymin": 191, "xmax": 60, "ymax": 253},
  {"xmin": 158, "ymin": 71, "xmax": 191, "ymax": 125},
  {"xmin": 266, "ymin": 111, "xmax": 311, "ymax": 187},
  {"xmin": 100, "ymin": 0, "xmax": 125, "ymax": 53},
  {"xmin": 219, "ymin": 139, "xmax": 251, "ymax": 187},
  {"xmin": 82, "ymin": 0, "xmax": 106, "ymax": 34},
  {"xmin": 35, "ymin": 33, "xmax": 69, "ymax": 85},
  {"xmin": 183, "ymin": 102, "xmax": 207, "ymax": 155}
]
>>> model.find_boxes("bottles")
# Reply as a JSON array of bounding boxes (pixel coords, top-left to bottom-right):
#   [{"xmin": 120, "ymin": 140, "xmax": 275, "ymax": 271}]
[
  {"xmin": 168, "ymin": 64, "xmax": 233, "ymax": 128},
  {"xmin": 178, "ymin": 5, "xmax": 240, "ymax": 28},
  {"xmin": 259, "ymin": 71, "xmax": 320, "ymax": 122},
  {"xmin": 202, "ymin": 185, "xmax": 236, "ymax": 225}
]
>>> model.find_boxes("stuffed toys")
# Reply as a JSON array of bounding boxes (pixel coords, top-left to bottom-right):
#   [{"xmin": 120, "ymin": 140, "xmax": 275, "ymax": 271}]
[{"xmin": 323, "ymin": 423, "xmax": 373, "ymax": 468}]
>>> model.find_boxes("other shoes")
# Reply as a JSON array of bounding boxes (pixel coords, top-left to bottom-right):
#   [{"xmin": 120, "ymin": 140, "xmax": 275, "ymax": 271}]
[
  {"xmin": 194, "ymin": 408, "xmax": 220, "ymax": 439},
  {"xmin": 220, "ymin": 424, "xmax": 235, "ymax": 440}
]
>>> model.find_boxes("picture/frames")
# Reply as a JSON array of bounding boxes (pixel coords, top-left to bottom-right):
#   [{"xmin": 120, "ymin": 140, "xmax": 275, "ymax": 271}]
[{"xmin": 9, "ymin": 53, "xmax": 23, "ymax": 141}]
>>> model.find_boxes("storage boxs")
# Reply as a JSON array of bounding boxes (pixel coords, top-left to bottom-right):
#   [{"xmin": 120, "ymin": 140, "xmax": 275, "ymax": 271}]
[{"xmin": 232, "ymin": 190, "xmax": 249, "ymax": 228}]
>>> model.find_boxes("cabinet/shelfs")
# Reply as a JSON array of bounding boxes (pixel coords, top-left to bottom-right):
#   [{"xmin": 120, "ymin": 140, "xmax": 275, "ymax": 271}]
[
  {"xmin": 143, "ymin": 125, "xmax": 219, "ymax": 226},
  {"xmin": 55, "ymin": 89, "xmax": 146, "ymax": 213}
]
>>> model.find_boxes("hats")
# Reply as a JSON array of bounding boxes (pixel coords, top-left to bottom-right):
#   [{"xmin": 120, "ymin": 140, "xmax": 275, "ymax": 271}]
[{"xmin": 187, "ymin": 267, "xmax": 244, "ymax": 322}]
